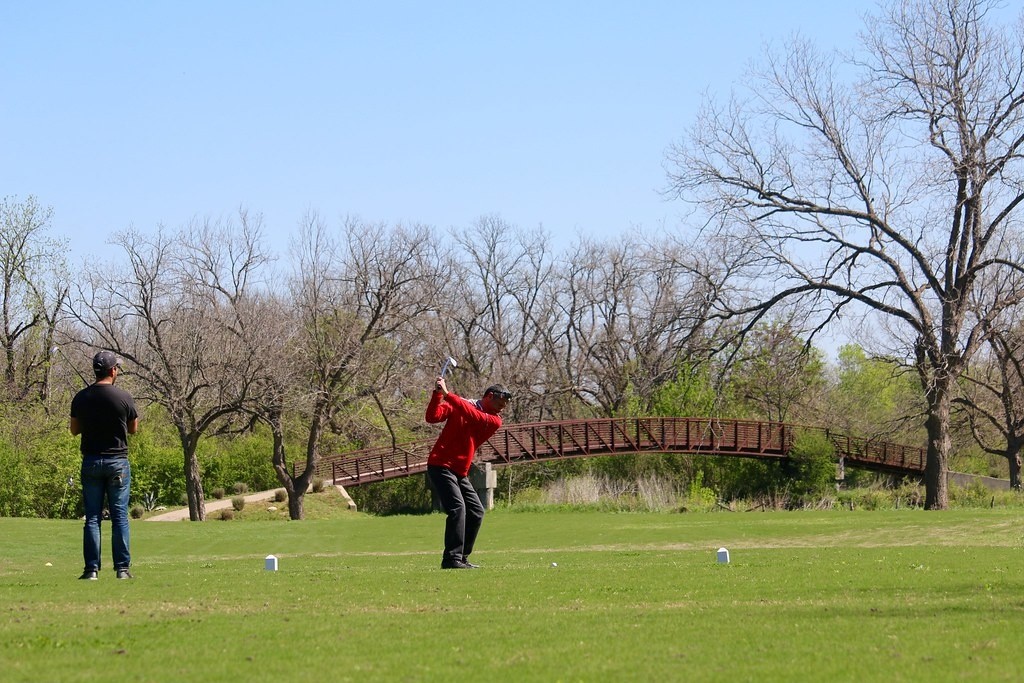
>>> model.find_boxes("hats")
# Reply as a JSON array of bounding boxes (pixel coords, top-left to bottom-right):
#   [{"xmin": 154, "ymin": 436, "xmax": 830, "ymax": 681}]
[{"xmin": 94, "ymin": 351, "xmax": 124, "ymax": 375}]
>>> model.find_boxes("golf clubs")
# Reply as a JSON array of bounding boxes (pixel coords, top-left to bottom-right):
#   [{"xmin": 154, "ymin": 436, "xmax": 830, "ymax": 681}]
[{"xmin": 437, "ymin": 356, "xmax": 457, "ymax": 389}]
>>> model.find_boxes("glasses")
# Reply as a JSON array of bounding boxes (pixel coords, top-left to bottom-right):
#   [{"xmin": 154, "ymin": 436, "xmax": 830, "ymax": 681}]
[{"xmin": 492, "ymin": 390, "xmax": 512, "ymax": 400}]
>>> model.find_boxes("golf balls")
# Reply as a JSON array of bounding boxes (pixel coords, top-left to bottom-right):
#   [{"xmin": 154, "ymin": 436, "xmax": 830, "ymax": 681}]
[
  {"xmin": 552, "ymin": 562, "xmax": 558, "ymax": 566},
  {"xmin": 45, "ymin": 562, "xmax": 52, "ymax": 566}
]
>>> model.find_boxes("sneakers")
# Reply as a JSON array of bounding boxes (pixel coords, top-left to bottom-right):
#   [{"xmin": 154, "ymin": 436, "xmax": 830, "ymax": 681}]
[
  {"xmin": 117, "ymin": 568, "xmax": 133, "ymax": 578},
  {"xmin": 442, "ymin": 558, "xmax": 474, "ymax": 568},
  {"xmin": 461, "ymin": 557, "xmax": 480, "ymax": 568},
  {"xmin": 79, "ymin": 569, "xmax": 98, "ymax": 580}
]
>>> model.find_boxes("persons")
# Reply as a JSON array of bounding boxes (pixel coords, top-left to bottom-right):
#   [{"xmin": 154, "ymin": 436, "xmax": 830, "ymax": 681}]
[
  {"xmin": 425, "ymin": 377, "xmax": 512, "ymax": 568},
  {"xmin": 70, "ymin": 350, "xmax": 139, "ymax": 580}
]
